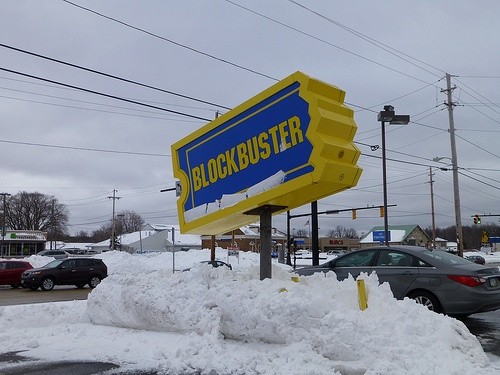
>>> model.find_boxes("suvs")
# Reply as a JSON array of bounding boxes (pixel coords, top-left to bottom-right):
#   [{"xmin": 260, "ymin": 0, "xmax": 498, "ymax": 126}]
[
  {"xmin": 0, "ymin": 259, "xmax": 35, "ymax": 289},
  {"xmin": 20, "ymin": 255, "xmax": 109, "ymax": 292}
]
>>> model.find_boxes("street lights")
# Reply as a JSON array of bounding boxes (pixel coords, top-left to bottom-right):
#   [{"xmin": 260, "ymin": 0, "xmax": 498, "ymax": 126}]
[
  {"xmin": 377, "ymin": 105, "xmax": 411, "ymax": 247},
  {"xmin": 433, "ymin": 156, "xmax": 463, "ymax": 258}
]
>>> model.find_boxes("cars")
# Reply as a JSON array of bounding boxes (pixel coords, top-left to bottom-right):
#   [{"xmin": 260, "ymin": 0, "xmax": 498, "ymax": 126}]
[
  {"xmin": 289, "ymin": 245, "xmax": 500, "ymax": 322},
  {"xmin": 271, "ymin": 248, "xmax": 359, "ymax": 259},
  {"xmin": 462, "ymin": 252, "xmax": 486, "ymax": 265},
  {"xmin": 35, "ymin": 247, "xmax": 100, "ymax": 260}
]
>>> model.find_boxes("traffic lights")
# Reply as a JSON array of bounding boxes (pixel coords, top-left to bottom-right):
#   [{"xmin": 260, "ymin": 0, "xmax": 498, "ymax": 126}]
[
  {"xmin": 474, "ymin": 217, "xmax": 477, "ymax": 224},
  {"xmin": 477, "ymin": 218, "xmax": 481, "ymax": 224}
]
